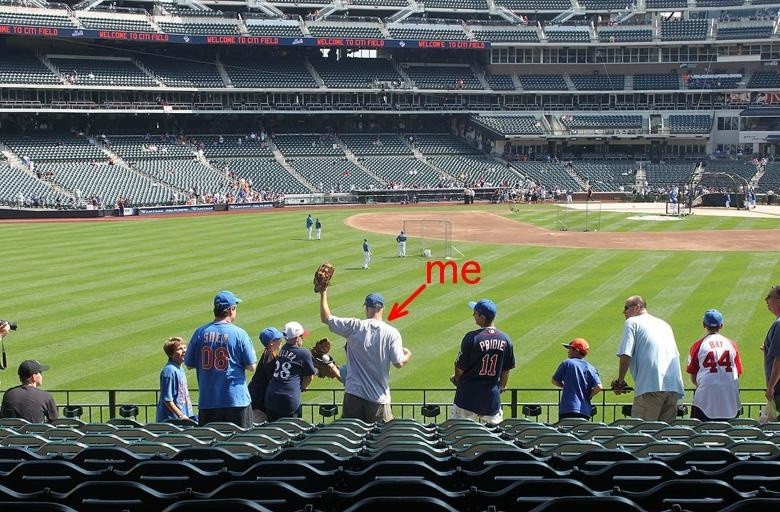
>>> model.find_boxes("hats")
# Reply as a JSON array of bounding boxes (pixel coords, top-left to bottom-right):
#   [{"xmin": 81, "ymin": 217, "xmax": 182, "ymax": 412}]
[
  {"xmin": 18, "ymin": 360, "xmax": 49, "ymax": 376},
  {"xmin": 469, "ymin": 299, "xmax": 496, "ymax": 316},
  {"xmin": 561, "ymin": 338, "xmax": 589, "ymax": 354},
  {"xmin": 704, "ymin": 310, "xmax": 723, "ymax": 328},
  {"xmin": 214, "ymin": 291, "xmax": 242, "ymax": 306},
  {"xmin": 362, "ymin": 293, "xmax": 384, "ymax": 308},
  {"xmin": 259, "ymin": 321, "xmax": 308, "ymax": 346}
]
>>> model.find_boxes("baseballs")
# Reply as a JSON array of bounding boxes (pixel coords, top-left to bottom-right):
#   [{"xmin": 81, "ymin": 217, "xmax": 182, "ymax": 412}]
[{"xmin": 322, "ymin": 354, "xmax": 330, "ymax": 362}]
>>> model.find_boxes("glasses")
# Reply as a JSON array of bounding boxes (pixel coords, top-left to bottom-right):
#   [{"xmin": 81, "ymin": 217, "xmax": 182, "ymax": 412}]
[{"xmin": 625, "ymin": 305, "xmax": 636, "ymax": 310}]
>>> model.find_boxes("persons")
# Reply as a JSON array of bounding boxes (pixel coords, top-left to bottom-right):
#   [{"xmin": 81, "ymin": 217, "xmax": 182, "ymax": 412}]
[
  {"xmin": 610, "ymin": 295, "xmax": 686, "ymax": 423},
  {"xmin": 0, "ymin": 319, "xmax": 17, "ymax": 370},
  {"xmin": 327, "ymin": 342, "xmax": 347, "ymax": 385},
  {"xmin": 685, "ymin": 308, "xmax": 743, "ymax": 420},
  {"xmin": 759, "ymin": 283, "xmax": 780, "ymax": 422},
  {"xmin": 248, "ymin": 325, "xmax": 285, "ymax": 424},
  {"xmin": 184, "ymin": 290, "xmax": 258, "ymax": 427},
  {"xmin": 0, "ymin": 357, "xmax": 61, "ymax": 422},
  {"xmin": 315, "ymin": 218, "xmax": 322, "ymax": 240},
  {"xmin": 449, "ymin": 298, "xmax": 515, "ymax": 426},
  {"xmin": 396, "ymin": 230, "xmax": 407, "ymax": 258},
  {"xmin": 313, "ymin": 261, "xmax": 412, "ymax": 426},
  {"xmin": 155, "ymin": 333, "xmax": 196, "ymax": 422},
  {"xmin": 306, "ymin": 212, "xmax": 313, "ymax": 240},
  {"xmin": 362, "ymin": 238, "xmax": 372, "ymax": 269},
  {"xmin": 1, "ymin": 0, "xmax": 780, "ymax": 216},
  {"xmin": 551, "ymin": 337, "xmax": 604, "ymax": 422},
  {"xmin": 263, "ymin": 319, "xmax": 320, "ymax": 419}
]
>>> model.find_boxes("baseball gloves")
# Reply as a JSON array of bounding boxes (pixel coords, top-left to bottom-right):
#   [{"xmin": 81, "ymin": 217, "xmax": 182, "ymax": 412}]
[
  {"xmin": 313, "ymin": 263, "xmax": 334, "ymax": 293},
  {"xmin": 311, "ymin": 338, "xmax": 338, "ymax": 379}
]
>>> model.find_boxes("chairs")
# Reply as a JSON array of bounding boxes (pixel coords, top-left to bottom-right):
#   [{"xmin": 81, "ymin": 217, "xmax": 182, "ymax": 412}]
[
  {"xmin": 1, "ymin": 416, "xmax": 780, "ymax": 512},
  {"xmin": 0, "ymin": 0, "xmax": 779, "ymax": 211}
]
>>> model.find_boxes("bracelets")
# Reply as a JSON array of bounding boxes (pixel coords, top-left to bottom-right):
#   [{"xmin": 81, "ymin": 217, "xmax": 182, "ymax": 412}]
[{"xmin": 179, "ymin": 413, "xmax": 185, "ymax": 420}]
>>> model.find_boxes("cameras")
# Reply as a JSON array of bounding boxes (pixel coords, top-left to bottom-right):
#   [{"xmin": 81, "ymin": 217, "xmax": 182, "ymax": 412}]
[{"xmin": 0, "ymin": 320, "xmax": 17, "ymax": 331}]
